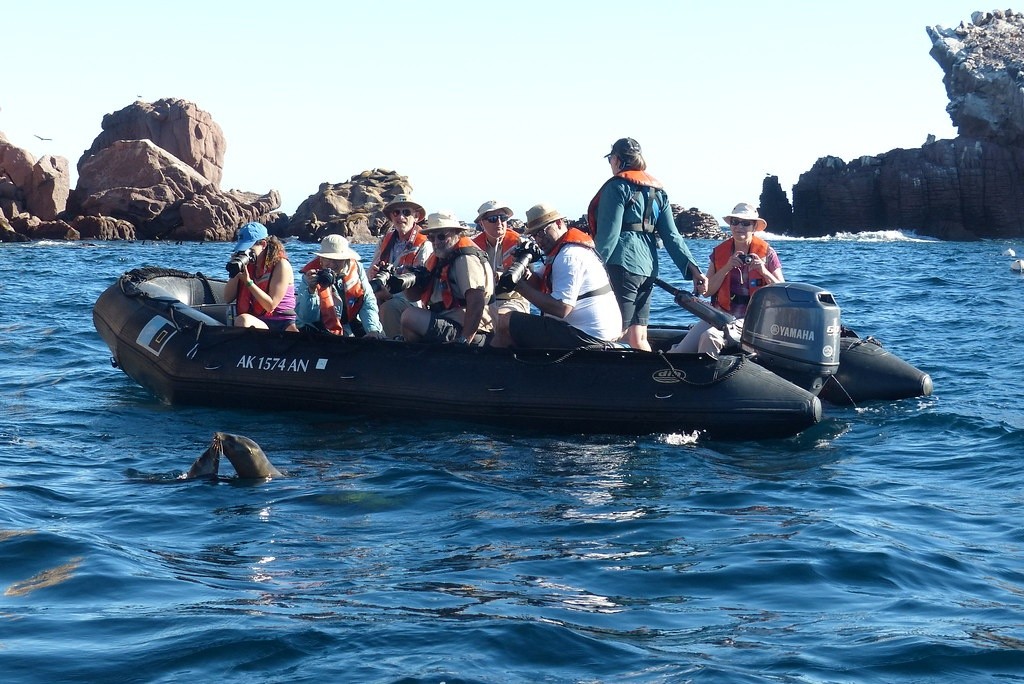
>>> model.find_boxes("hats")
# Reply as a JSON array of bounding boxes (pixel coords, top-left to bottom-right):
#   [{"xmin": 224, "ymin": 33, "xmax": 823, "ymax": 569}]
[
  {"xmin": 232, "ymin": 222, "xmax": 268, "ymax": 252},
  {"xmin": 381, "ymin": 194, "xmax": 425, "ymax": 223},
  {"xmin": 474, "ymin": 200, "xmax": 514, "ymax": 224},
  {"xmin": 604, "ymin": 137, "xmax": 642, "ymax": 157},
  {"xmin": 418, "ymin": 211, "xmax": 470, "ymax": 234},
  {"xmin": 524, "ymin": 201, "xmax": 566, "ymax": 231},
  {"xmin": 312, "ymin": 234, "xmax": 362, "ymax": 262},
  {"xmin": 721, "ymin": 202, "xmax": 767, "ymax": 232}
]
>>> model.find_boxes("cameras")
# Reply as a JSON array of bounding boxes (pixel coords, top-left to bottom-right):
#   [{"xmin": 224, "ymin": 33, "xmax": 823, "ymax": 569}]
[
  {"xmin": 226, "ymin": 248, "xmax": 257, "ymax": 278},
  {"xmin": 498, "ymin": 241, "xmax": 542, "ymax": 293},
  {"xmin": 316, "ymin": 268, "xmax": 337, "ymax": 287},
  {"xmin": 739, "ymin": 254, "xmax": 753, "ymax": 264},
  {"xmin": 369, "ymin": 264, "xmax": 394, "ymax": 292},
  {"xmin": 387, "ymin": 266, "xmax": 431, "ymax": 294}
]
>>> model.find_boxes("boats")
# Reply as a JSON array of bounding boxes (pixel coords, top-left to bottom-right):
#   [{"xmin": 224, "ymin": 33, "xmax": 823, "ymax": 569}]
[{"xmin": 92, "ymin": 266, "xmax": 936, "ymax": 445}]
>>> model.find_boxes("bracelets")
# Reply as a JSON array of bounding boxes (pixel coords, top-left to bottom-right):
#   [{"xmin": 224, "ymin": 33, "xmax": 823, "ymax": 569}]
[{"xmin": 245, "ymin": 280, "xmax": 254, "ymax": 288}]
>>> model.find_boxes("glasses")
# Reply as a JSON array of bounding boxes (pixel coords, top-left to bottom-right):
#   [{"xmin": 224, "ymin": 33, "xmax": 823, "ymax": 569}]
[
  {"xmin": 527, "ymin": 223, "xmax": 554, "ymax": 241},
  {"xmin": 480, "ymin": 213, "xmax": 508, "ymax": 223},
  {"xmin": 391, "ymin": 209, "xmax": 416, "ymax": 219},
  {"xmin": 730, "ymin": 218, "xmax": 755, "ymax": 226},
  {"xmin": 426, "ymin": 232, "xmax": 452, "ymax": 242}
]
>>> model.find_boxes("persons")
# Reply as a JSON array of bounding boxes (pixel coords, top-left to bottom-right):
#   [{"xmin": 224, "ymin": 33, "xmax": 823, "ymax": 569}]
[
  {"xmin": 468, "ymin": 198, "xmax": 530, "ymax": 333},
  {"xmin": 365, "ymin": 193, "xmax": 434, "ymax": 342},
  {"xmin": 295, "ymin": 233, "xmax": 386, "ymax": 339},
  {"xmin": 223, "ymin": 224, "xmax": 301, "ymax": 333},
  {"xmin": 668, "ymin": 204, "xmax": 784, "ymax": 356},
  {"xmin": 588, "ymin": 137, "xmax": 709, "ymax": 352},
  {"xmin": 398, "ymin": 209, "xmax": 497, "ymax": 347},
  {"xmin": 497, "ymin": 203, "xmax": 622, "ymax": 349}
]
